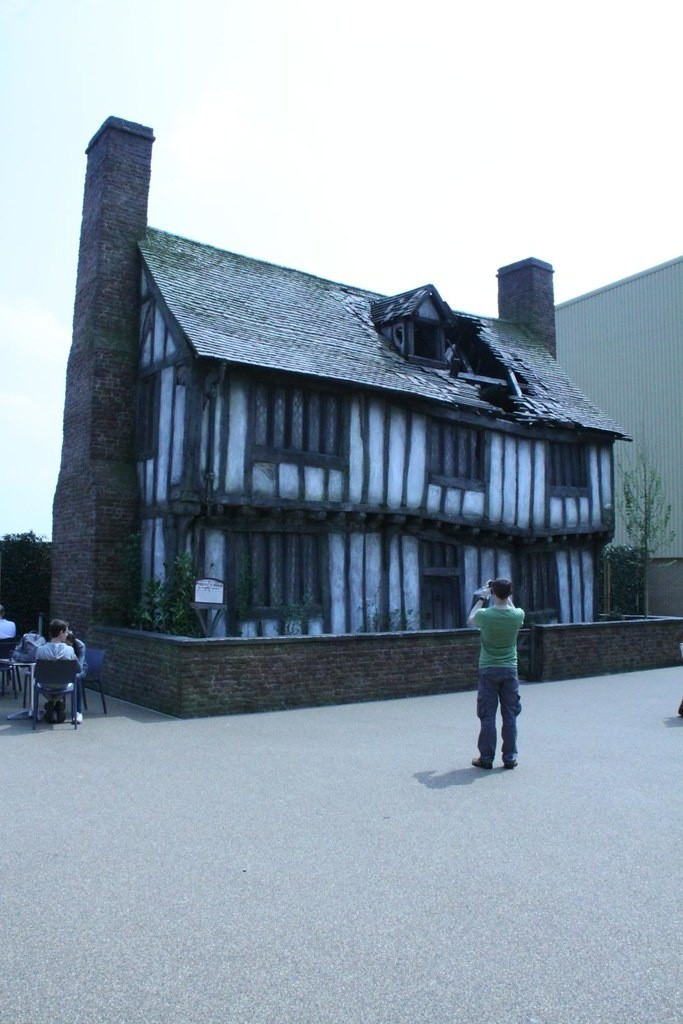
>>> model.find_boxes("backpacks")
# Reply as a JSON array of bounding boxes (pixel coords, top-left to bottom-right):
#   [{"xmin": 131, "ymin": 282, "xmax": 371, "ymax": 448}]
[{"xmin": 13, "ymin": 630, "xmax": 46, "ymax": 663}]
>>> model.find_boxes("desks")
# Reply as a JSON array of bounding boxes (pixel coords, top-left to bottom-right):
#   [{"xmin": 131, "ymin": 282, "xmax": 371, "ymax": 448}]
[
  {"xmin": 0, "ymin": 658, "xmax": 15, "ymax": 663},
  {"xmin": 6, "ymin": 662, "xmax": 36, "ymax": 721}
]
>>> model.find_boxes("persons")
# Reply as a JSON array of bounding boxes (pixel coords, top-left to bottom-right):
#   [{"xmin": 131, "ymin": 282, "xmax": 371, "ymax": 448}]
[
  {"xmin": 465, "ymin": 577, "xmax": 526, "ymax": 773},
  {"xmin": 0, "ymin": 605, "xmax": 16, "ymax": 643},
  {"xmin": 35, "ymin": 618, "xmax": 88, "ymax": 724}
]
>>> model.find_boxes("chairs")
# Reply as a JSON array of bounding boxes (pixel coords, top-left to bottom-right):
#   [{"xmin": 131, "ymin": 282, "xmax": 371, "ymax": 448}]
[
  {"xmin": 0, "ymin": 643, "xmax": 22, "ymax": 696},
  {"xmin": 83, "ymin": 650, "xmax": 108, "ymax": 713},
  {"xmin": 34, "ymin": 660, "xmax": 78, "ymax": 732}
]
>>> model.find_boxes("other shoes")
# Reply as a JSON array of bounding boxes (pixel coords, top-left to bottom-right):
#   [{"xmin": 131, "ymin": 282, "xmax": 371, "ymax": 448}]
[
  {"xmin": 505, "ymin": 760, "xmax": 517, "ymax": 769},
  {"xmin": 77, "ymin": 712, "xmax": 83, "ymax": 724},
  {"xmin": 472, "ymin": 758, "xmax": 492, "ymax": 769},
  {"xmin": 44, "ymin": 700, "xmax": 66, "ymax": 723}
]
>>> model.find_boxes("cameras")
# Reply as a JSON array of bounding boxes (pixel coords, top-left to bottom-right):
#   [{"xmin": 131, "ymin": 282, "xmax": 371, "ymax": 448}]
[{"xmin": 489, "ymin": 581, "xmax": 494, "ymax": 587}]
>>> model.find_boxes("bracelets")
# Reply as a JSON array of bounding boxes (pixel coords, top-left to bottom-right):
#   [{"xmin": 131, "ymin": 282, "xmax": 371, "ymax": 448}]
[{"xmin": 478, "ymin": 595, "xmax": 485, "ymax": 601}]
